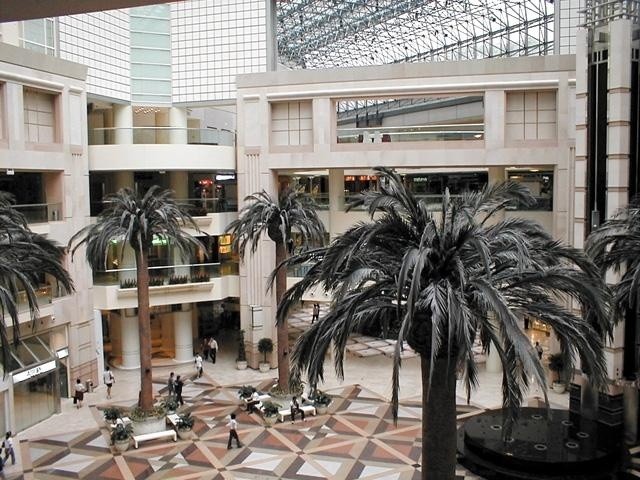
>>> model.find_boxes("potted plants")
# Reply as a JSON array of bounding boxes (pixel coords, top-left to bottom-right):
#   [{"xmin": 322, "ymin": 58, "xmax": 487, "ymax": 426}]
[
  {"xmin": 161, "ymin": 394, "xmax": 194, "ymax": 440},
  {"xmin": 236, "ymin": 329, "xmax": 272, "ymax": 373},
  {"xmin": 317, "ymin": 390, "xmax": 331, "ymax": 415},
  {"xmin": 104, "ymin": 406, "xmax": 134, "ymax": 450},
  {"xmin": 238, "ymin": 384, "xmax": 257, "ymax": 408},
  {"xmin": 549, "ymin": 353, "xmax": 568, "ymax": 394}
]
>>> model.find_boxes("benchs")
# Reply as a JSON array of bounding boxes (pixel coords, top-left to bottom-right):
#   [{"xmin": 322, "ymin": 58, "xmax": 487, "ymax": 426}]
[
  {"xmin": 134, "ymin": 429, "xmax": 177, "ymax": 449},
  {"xmin": 244, "ymin": 395, "xmax": 317, "ymax": 422}
]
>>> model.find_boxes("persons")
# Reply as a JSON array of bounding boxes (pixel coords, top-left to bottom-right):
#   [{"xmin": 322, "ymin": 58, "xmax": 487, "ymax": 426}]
[
  {"xmin": 2, "ymin": 431, "xmax": 17, "ymax": 465},
  {"xmin": 244, "ymin": 388, "xmax": 261, "ymax": 415},
  {"xmin": 109, "ymin": 416, "xmax": 125, "ymax": 446},
  {"xmin": 194, "ymin": 353, "xmax": 203, "ymax": 377},
  {"xmin": 0, "ymin": 448, "xmax": 7, "ymax": 480},
  {"xmin": 289, "ymin": 396, "xmax": 307, "ymax": 424},
  {"xmin": 200, "ymin": 338, "xmax": 209, "ymax": 361},
  {"xmin": 226, "ymin": 413, "xmax": 242, "ymax": 450},
  {"xmin": 167, "ymin": 372, "xmax": 176, "ymax": 397},
  {"xmin": 103, "ymin": 366, "xmax": 116, "ymax": 399},
  {"xmin": 173, "ymin": 375, "xmax": 186, "ymax": 406},
  {"xmin": 75, "ymin": 379, "xmax": 86, "ymax": 409},
  {"xmin": 272, "ymin": 379, "xmax": 279, "ymax": 387},
  {"xmin": 535, "ymin": 341, "xmax": 543, "ymax": 360},
  {"xmin": 208, "ymin": 336, "xmax": 218, "ymax": 364},
  {"xmin": 310, "ymin": 304, "xmax": 318, "ymax": 324}
]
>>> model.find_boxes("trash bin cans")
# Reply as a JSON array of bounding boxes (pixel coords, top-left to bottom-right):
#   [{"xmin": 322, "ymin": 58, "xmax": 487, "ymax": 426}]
[{"xmin": 86, "ymin": 379, "xmax": 93, "ymax": 393}]
